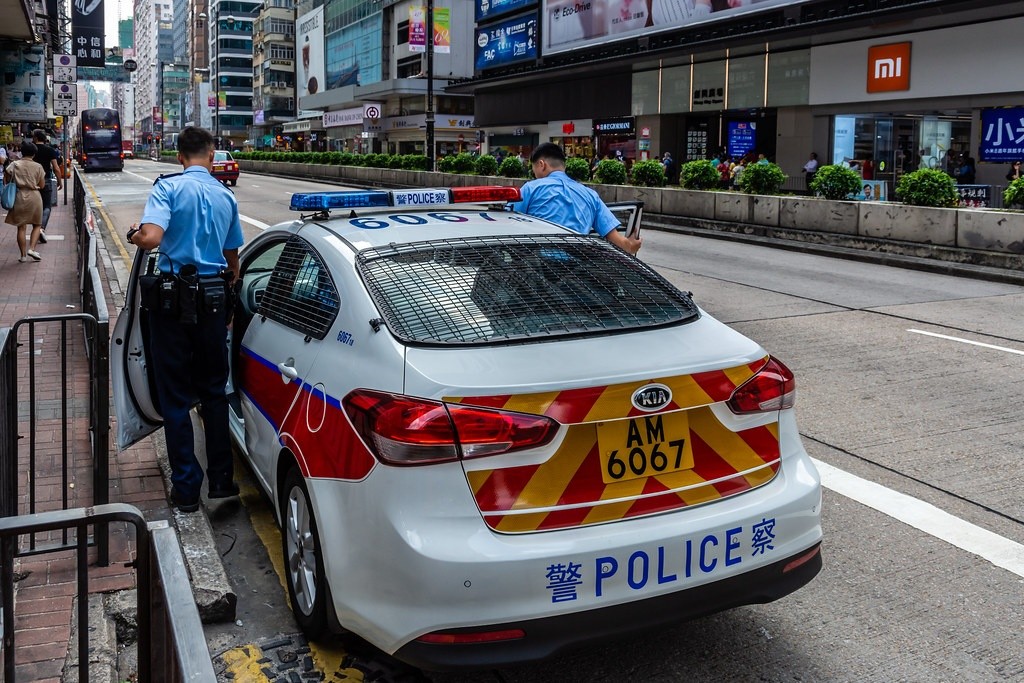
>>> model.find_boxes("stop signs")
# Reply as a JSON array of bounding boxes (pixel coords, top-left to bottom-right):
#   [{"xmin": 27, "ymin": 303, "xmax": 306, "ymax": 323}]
[{"xmin": 458, "ymin": 133, "xmax": 464, "ymax": 144}]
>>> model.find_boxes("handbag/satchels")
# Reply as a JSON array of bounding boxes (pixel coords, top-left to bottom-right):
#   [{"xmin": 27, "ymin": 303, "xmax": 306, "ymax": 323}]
[{"xmin": 0, "ymin": 161, "xmax": 17, "ymax": 210}]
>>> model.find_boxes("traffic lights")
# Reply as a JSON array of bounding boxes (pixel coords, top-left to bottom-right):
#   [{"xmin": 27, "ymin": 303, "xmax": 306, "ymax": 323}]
[
  {"xmin": 147, "ymin": 134, "xmax": 152, "ymax": 145},
  {"xmin": 155, "ymin": 135, "xmax": 160, "ymax": 147},
  {"xmin": 273, "ymin": 125, "xmax": 284, "ymax": 143}
]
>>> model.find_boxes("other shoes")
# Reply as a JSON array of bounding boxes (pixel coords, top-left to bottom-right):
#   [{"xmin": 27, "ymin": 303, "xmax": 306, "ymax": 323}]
[
  {"xmin": 40, "ymin": 228, "xmax": 48, "ymax": 243},
  {"xmin": 18, "ymin": 250, "xmax": 42, "ymax": 262}
]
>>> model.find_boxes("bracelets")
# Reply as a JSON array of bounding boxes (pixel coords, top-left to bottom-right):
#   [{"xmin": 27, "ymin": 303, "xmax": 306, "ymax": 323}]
[{"xmin": 126, "ymin": 228, "xmax": 139, "ymax": 245}]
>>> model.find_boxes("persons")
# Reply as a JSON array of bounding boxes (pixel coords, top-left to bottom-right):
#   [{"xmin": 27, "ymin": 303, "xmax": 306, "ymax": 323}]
[
  {"xmin": 863, "ymin": 184, "xmax": 873, "ymax": 201},
  {"xmin": 4, "ymin": 142, "xmax": 46, "ymax": 262},
  {"xmin": 843, "ymin": 149, "xmax": 977, "ymax": 183},
  {"xmin": 1005, "ymin": 159, "xmax": 1024, "ymax": 184},
  {"xmin": 801, "ymin": 152, "xmax": 818, "ymax": 196},
  {"xmin": 127, "ymin": 126, "xmax": 245, "ymax": 512},
  {"xmin": 497, "ymin": 151, "xmax": 526, "ymax": 166},
  {"xmin": 0, "ymin": 140, "xmax": 61, "ymax": 204},
  {"xmin": 589, "ymin": 151, "xmax": 673, "ymax": 184},
  {"xmin": 710, "ymin": 153, "xmax": 769, "ymax": 192},
  {"xmin": 508, "ymin": 143, "xmax": 641, "ymax": 256},
  {"xmin": 29, "ymin": 129, "xmax": 65, "ymax": 244}
]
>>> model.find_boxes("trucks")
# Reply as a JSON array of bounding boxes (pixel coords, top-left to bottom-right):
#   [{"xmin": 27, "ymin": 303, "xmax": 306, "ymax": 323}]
[{"xmin": 122, "ymin": 140, "xmax": 133, "ymax": 159}]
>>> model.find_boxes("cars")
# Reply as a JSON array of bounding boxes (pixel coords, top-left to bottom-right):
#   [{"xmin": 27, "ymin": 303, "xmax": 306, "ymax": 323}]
[
  {"xmin": 206, "ymin": 150, "xmax": 240, "ymax": 186},
  {"xmin": 110, "ymin": 184, "xmax": 824, "ymax": 669}
]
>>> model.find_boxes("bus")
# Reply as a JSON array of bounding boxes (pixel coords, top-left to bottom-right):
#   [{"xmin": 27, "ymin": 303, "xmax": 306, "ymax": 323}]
[{"xmin": 74, "ymin": 108, "xmax": 124, "ymax": 174}]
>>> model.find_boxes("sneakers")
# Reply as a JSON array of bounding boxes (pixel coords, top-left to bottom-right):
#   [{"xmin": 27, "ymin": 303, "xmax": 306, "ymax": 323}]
[
  {"xmin": 208, "ymin": 480, "xmax": 241, "ymax": 498},
  {"xmin": 170, "ymin": 487, "xmax": 200, "ymax": 513}
]
